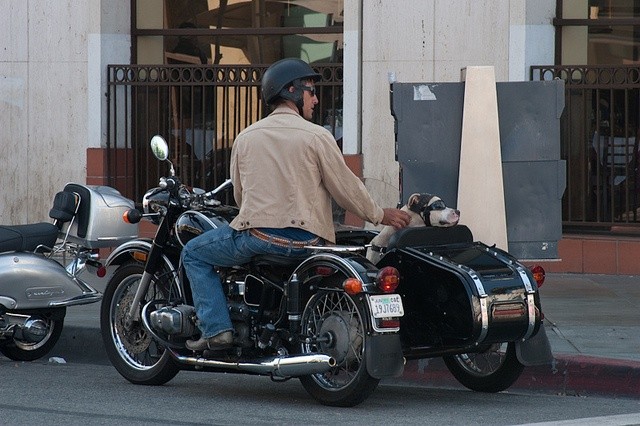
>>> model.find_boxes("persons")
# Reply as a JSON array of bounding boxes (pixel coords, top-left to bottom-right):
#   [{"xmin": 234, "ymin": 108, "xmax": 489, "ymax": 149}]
[{"xmin": 180, "ymin": 56, "xmax": 413, "ymax": 353}]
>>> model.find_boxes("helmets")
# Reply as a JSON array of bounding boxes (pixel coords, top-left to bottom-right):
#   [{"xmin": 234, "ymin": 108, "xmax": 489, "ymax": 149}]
[{"xmin": 261, "ymin": 58, "xmax": 322, "ymax": 104}]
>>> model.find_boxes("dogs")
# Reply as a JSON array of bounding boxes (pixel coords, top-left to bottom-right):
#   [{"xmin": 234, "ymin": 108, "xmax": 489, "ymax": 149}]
[{"xmin": 365, "ymin": 192, "xmax": 461, "ymax": 266}]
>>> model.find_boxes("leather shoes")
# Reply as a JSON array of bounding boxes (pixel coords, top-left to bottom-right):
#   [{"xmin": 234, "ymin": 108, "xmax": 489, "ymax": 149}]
[{"xmin": 184, "ymin": 332, "xmax": 234, "ymax": 351}]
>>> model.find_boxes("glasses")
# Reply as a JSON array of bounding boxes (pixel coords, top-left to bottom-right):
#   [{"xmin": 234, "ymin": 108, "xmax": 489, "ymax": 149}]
[
  {"xmin": 295, "ymin": 86, "xmax": 317, "ymax": 96},
  {"xmin": 425, "ymin": 200, "xmax": 445, "ymax": 210}
]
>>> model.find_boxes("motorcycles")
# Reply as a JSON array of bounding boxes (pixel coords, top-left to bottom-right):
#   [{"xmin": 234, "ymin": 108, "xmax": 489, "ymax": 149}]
[
  {"xmin": 98, "ymin": 130, "xmax": 555, "ymax": 406},
  {"xmin": 0, "ymin": 181, "xmax": 142, "ymax": 363}
]
